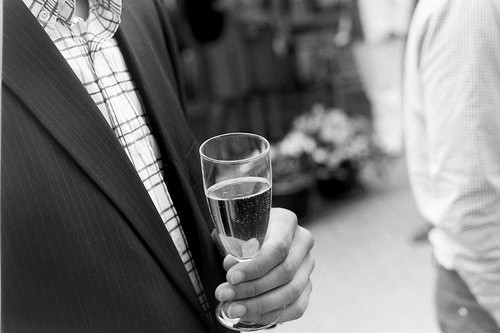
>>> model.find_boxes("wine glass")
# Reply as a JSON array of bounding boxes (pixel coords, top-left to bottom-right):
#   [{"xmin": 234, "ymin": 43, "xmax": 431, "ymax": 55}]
[{"xmin": 199, "ymin": 133, "xmax": 285, "ymax": 331}]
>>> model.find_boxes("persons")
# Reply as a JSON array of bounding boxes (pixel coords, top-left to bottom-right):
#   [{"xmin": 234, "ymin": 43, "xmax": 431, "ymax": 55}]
[
  {"xmin": 404, "ymin": 0, "xmax": 500, "ymax": 333},
  {"xmin": 1, "ymin": 0, "xmax": 315, "ymax": 333}
]
